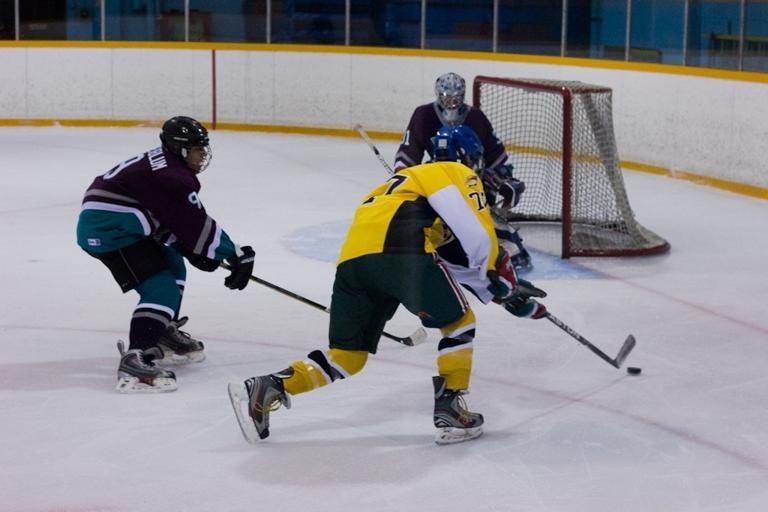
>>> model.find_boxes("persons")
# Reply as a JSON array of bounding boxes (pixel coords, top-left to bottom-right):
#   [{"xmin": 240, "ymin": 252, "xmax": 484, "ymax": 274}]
[
  {"xmin": 393, "ymin": 72, "xmax": 531, "ymax": 271},
  {"xmin": 75, "ymin": 115, "xmax": 254, "ymax": 388},
  {"xmin": 244, "ymin": 123, "xmax": 548, "ymax": 440}
]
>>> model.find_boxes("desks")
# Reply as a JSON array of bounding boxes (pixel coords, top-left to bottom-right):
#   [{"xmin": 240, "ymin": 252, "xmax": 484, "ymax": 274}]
[{"xmin": 709, "ymin": 33, "xmax": 768, "ymax": 70}]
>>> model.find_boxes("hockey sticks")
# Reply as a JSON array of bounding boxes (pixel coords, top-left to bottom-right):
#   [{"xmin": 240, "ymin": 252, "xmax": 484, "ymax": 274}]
[
  {"xmin": 547, "ymin": 314, "xmax": 635, "ymax": 368},
  {"xmin": 219, "ymin": 263, "xmax": 427, "ymax": 345}
]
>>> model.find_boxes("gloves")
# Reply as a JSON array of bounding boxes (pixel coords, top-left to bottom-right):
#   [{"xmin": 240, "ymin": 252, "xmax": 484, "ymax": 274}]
[
  {"xmin": 225, "ymin": 246, "xmax": 255, "ymax": 290},
  {"xmin": 486, "ymin": 245, "xmax": 547, "ymax": 319}
]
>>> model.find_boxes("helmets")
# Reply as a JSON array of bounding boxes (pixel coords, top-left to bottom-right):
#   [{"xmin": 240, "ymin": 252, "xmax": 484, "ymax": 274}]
[
  {"xmin": 433, "ymin": 123, "xmax": 484, "ymax": 173},
  {"xmin": 160, "ymin": 115, "xmax": 212, "ymax": 172},
  {"xmin": 435, "ymin": 72, "xmax": 465, "ymax": 109}
]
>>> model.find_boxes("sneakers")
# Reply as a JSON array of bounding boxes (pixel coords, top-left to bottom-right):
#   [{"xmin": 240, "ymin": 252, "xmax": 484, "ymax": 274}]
[
  {"xmin": 118, "ymin": 316, "xmax": 203, "ymax": 385},
  {"xmin": 433, "ymin": 376, "xmax": 483, "ymax": 428},
  {"xmin": 245, "ymin": 366, "xmax": 296, "ymax": 439}
]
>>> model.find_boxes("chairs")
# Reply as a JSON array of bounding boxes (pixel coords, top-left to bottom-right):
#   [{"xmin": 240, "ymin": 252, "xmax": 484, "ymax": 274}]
[{"xmin": 604, "ymin": 44, "xmax": 662, "ymax": 66}]
